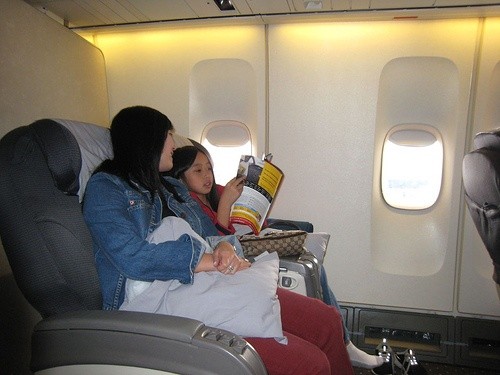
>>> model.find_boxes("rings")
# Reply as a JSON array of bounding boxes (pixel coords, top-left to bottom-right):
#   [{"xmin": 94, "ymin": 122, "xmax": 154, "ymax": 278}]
[{"xmin": 227, "ymin": 265, "xmax": 234, "ymax": 271}]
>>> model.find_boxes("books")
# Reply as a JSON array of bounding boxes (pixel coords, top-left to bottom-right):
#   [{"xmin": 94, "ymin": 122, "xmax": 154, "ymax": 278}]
[{"xmin": 226, "ymin": 153, "xmax": 286, "ymax": 237}]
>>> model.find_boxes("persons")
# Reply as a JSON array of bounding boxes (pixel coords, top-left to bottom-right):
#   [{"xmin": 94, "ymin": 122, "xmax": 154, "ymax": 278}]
[
  {"xmin": 172, "ymin": 144, "xmax": 386, "ymax": 370},
  {"xmin": 82, "ymin": 105, "xmax": 359, "ymax": 375}
]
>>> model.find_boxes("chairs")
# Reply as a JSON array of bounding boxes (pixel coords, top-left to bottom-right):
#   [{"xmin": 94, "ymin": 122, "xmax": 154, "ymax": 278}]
[
  {"xmin": 0, "ymin": 118, "xmax": 321, "ymax": 375},
  {"xmin": 462, "ymin": 128, "xmax": 500, "ymax": 288}
]
